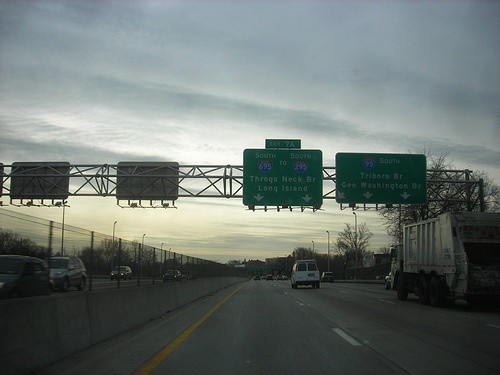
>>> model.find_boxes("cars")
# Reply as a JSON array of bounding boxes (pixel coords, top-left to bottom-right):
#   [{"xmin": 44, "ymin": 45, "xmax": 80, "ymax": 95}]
[
  {"xmin": 43, "ymin": 256, "xmax": 88, "ymax": 291},
  {"xmin": 110, "ymin": 265, "xmax": 133, "ymax": 281},
  {"xmin": 0, "ymin": 255, "xmax": 49, "ymax": 299},
  {"xmin": 163, "ymin": 270, "xmax": 182, "ymax": 281},
  {"xmin": 384, "ymin": 272, "xmax": 392, "ymax": 289},
  {"xmin": 253, "ymin": 274, "xmax": 289, "ymax": 280},
  {"xmin": 320, "ymin": 271, "xmax": 335, "ymax": 282}
]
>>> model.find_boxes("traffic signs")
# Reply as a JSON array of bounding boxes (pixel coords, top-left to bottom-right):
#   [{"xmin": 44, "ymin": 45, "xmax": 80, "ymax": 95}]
[
  {"xmin": 335, "ymin": 152, "xmax": 427, "ymax": 205},
  {"xmin": 242, "ymin": 148, "xmax": 323, "ymax": 207}
]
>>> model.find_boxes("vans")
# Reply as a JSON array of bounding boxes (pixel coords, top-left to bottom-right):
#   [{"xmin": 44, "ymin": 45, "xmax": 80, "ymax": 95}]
[{"xmin": 290, "ymin": 260, "xmax": 320, "ymax": 288}]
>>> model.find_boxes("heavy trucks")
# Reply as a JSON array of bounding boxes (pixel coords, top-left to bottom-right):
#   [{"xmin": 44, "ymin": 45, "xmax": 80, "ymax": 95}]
[{"xmin": 389, "ymin": 211, "xmax": 500, "ymax": 312}]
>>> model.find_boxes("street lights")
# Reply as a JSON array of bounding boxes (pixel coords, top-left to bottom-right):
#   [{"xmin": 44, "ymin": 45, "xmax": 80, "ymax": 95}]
[
  {"xmin": 352, "ymin": 211, "xmax": 358, "ymax": 280},
  {"xmin": 326, "ymin": 230, "xmax": 330, "ymax": 272},
  {"xmin": 141, "ymin": 234, "xmax": 146, "ymax": 276},
  {"xmin": 160, "ymin": 243, "xmax": 164, "ymax": 277},
  {"xmin": 312, "ymin": 241, "xmax": 315, "ymax": 260},
  {"xmin": 61, "ymin": 200, "xmax": 69, "ymax": 256},
  {"xmin": 113, "ymin": 220, "xmax": 118, "ymax": 265}
]
